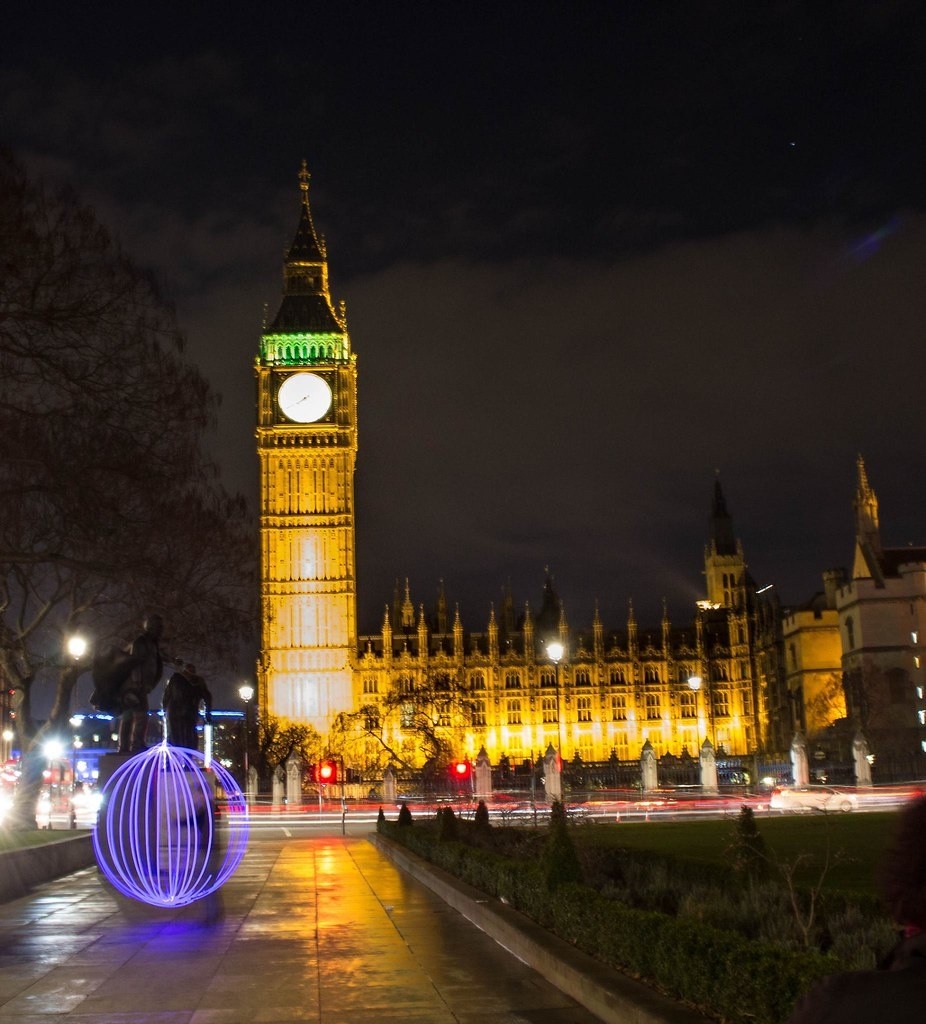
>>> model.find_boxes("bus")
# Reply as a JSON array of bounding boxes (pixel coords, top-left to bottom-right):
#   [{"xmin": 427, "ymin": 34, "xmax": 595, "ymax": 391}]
[{"xmin": 67, "ymin": 712, "xmax": 120, "ymax": 789}]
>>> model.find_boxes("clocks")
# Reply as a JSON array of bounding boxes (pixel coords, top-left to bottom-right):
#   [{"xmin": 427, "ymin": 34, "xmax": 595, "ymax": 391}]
[{"xmin": 278, "ymin": 372, "xmax": 333, "ymax": 425}]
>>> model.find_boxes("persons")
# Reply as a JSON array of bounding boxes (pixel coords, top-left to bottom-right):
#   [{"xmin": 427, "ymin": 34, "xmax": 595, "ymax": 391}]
[
  {"xmin": 162, "ymin": 663, "xmax": 213, "ymax": 772},
  {"xmin": 118, "ymin": 614, "xmax": 184, "ymax": 754}
]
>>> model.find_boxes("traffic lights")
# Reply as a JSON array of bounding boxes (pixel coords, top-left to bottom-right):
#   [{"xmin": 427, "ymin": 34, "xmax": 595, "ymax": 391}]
[
  {"xmin": 457, "ymin": 755, "xmax": 469, "ymax": 777},
  {"xmin": 319, "ymin": 757, "xmax": 336, "ymax": 783}
]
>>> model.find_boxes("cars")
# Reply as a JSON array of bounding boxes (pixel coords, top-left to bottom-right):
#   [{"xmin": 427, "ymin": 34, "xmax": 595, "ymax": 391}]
[{"xmin": 770, "ymin": 782, "xmax": 859, "ymax": 813}]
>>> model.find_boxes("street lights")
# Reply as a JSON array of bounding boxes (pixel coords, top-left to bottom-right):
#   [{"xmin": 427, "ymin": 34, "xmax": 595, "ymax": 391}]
[
  {"xmin": 237, "ymin": 679, "xmax": 255, "ymax": 812},
  {"xmin": 67, "ymin": 635, "xmax": 86, "ymax": 830},
  {"xmin": 545, "ymin": 643, "xmax": 565, "ymax": 820},
  {"xmin": 687, "ymin": 673, "xmax": 702, "ymax": 795}
]
